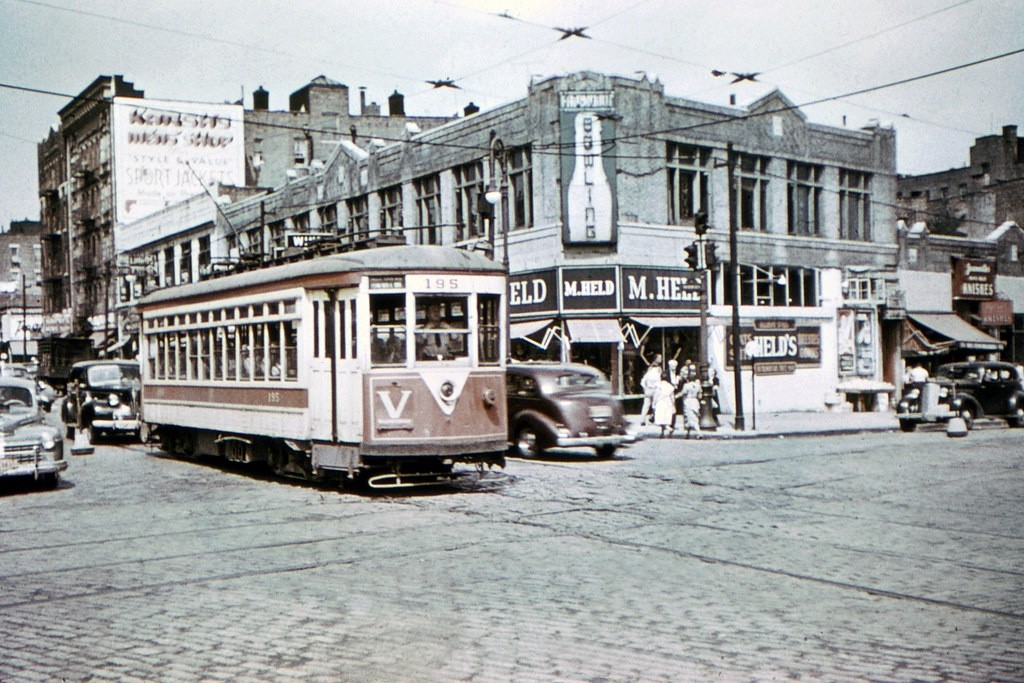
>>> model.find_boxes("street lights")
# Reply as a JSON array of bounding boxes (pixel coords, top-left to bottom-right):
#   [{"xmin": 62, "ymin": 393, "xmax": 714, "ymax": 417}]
[{"xmin": 486, "ymin": 137, "xmax": 513, "ymax": 360}]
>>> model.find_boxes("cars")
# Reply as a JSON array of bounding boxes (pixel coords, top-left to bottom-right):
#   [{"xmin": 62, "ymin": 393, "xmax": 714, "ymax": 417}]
[
  {"xmin": 0, "ymin": 379, "xmax": 69, "ymax": 478},
  {"xmin": 898, "ymin": 361, "xmax": 1024, "ymax": 430},
  {"xmin": 60, "ymin": 356, "xmax": 140, "ymax": 445},
  {"xmin": 504, "ymin": 364, "xmax": 643, "ymax": 459}
]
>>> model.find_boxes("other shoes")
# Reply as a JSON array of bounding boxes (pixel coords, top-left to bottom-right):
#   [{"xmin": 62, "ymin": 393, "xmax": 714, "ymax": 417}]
[
  {"xmin": 696, "ymin": 434, "xmax": 703, "ymax": 439},
  {"xmin": 656, "ymin": 434, "xmax": 664, "ymax": 438},
  {"xmin": 640, "ymin": 422, "xmax": 645, "ymax": 426},
  {"xmin": 668, "ymin": 428, "xmax": 674, "ymax": 438},
  {"xmin": 684, "ymin": 435, "xmax": 689, "ymax": 439}
]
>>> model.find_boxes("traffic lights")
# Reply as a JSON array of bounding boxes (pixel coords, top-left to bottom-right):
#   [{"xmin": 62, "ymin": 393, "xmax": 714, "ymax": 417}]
[
  {"xmin": 683, "ymin": 245, "xmax": 699, "ymax": 268},
  {"xmin": 704, "ymin": 240, "xmax": 721, "ymax": 266}
]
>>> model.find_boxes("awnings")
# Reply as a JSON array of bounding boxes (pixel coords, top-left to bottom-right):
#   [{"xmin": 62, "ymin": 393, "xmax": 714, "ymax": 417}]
[
  {"xmin": 630, "ymin": 316, "xmax": 732, "ymax": 342},
  {"xmin": 565, "ymin": 319, "xmax": 628, "ymax": 343},
  {"xmin": 909, "ymin": 310, "xmax": 1007, "ymax": 350},
  {"xmin": 509, "ymin": 318, "xmax": 554, "ymax": 341}
]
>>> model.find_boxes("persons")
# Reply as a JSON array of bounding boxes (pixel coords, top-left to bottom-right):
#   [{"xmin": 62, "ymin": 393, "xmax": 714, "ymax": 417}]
[
  {"xmin": 417, "ymin": 301, "xmax": 460, "ymax": 360},
  {"xmin": 1013, "ymin": 358, "xmax": 1024, "ymax": 388},
  {"xmin": 909, "ymin": 362, "xmax": 930, "ymax": 382},
  {"xmin": 384, "ymin": 335, "xmax": 406, "ymax": 363},
  {"xmin": 640, "ymin": 353, "xmax": 720, "ymax": 439}
]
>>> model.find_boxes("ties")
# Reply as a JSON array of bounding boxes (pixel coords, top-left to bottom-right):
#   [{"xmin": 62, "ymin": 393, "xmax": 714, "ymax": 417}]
[{"xmin": 433, "ymin": 323, "xmax": 442, "ymax": 347}]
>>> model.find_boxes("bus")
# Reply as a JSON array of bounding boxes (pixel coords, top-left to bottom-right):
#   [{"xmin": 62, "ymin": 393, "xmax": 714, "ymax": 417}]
[{"xmin": 138, "ymin": 244, "xmax": 507, "ymax": 489}]
